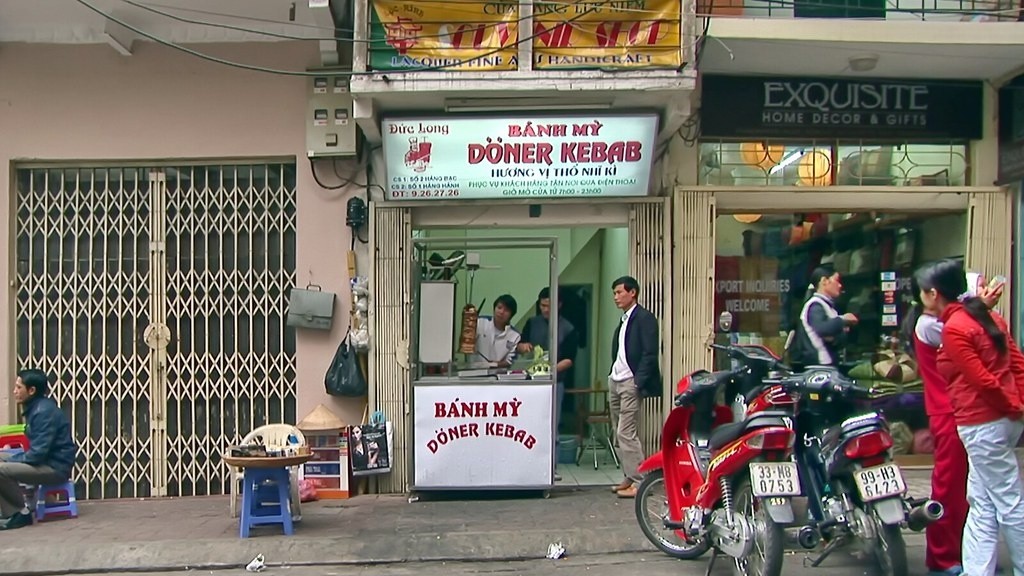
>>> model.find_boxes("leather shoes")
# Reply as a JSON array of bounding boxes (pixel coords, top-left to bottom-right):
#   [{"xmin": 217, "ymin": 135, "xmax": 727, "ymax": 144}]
[
  {"xmin": 0, "ymin": 506, "xmax": 33, "ymax": 530},
  {"xmin": 611, "ymin": 480, "xmax": 654, "ymax": 498}
]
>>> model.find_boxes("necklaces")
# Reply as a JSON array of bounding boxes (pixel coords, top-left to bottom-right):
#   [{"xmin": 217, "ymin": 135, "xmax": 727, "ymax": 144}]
[{"xmin": 920, "ymin": 312, "xmax": 936, "ymax": 318}]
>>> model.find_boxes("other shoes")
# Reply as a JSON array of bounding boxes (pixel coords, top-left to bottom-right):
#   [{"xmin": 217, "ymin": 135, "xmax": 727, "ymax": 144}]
[
  {"xmin": 555, "ymin": 472, "xmax": 562, "ymax": 480},
  {"xmin": 996, "ymin": 565, "xmax": 1004, "ymax": 573},
  {"xmin": 926, "ymin": 566, "xmax": 963, "ymax": 576}
]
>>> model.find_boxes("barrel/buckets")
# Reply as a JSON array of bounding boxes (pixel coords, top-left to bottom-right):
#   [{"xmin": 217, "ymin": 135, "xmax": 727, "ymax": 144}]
[{"xmin": 559, "ymin": 434, "xmax": 578, "ymax": 464}]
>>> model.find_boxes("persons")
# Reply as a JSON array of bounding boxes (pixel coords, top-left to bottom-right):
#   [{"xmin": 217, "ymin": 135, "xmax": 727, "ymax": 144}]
[
  {"xmin": 517, "ymin": 287, "xmax": 579, "ymax": 481},
  {"xmin": 903, "ymin": 259, "xmax": 1024, "ymax": 576},
  {"xmin": 0, "ymin": 369, "xmax": 76, "ymax": 531},
  {"xmin": 607, "ymin": 276, "xmax": 658, "ymax": 499},
  {"xmin": 789, "ymin": 264, "xmax": 859, "ymax": 373},
  {"xmin": 466, "ymin": 294, "xmax": 522, "ymax": 368}
]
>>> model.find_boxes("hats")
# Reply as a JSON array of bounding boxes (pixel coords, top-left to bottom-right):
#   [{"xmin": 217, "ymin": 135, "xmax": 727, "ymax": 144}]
[{"xmin": 295, "ymin": 403, "xmax": 346, "ymax": 430}]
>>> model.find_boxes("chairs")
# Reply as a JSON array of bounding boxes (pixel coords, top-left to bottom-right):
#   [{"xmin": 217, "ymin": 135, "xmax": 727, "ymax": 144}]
[{"xmin": 229, "ymin": 424, "xmax": 305, "ymax": 521}]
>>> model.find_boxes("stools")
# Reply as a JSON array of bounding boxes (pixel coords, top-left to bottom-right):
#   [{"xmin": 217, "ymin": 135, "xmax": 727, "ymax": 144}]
[
  {"xmin": 34, "ymin": 482, "xmax": 78, "ymax": 521},
  {"xmin": 577, "ymin": 412, "xmax": 620, "ymax": 470},
  {"xmin": 241, "ymin": 469, "xmax": 293, "ymax": 538}
]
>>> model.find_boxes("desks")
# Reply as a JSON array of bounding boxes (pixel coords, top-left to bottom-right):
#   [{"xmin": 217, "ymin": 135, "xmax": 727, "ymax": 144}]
[{"xmin": 563, "ymin": 388, "xmax": 622, "ymax": 466}]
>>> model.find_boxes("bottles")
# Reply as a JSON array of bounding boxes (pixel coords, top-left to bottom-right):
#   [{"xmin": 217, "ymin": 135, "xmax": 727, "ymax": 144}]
[{"xmin": 986, "ymin": 275, "xmax": 1005, "ymax": 295}]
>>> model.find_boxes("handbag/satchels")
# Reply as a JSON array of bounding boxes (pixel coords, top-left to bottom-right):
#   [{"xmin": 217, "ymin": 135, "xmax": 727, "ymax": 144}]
[
  {"xmin": 347, "ymin": 403, "xmax": 393, "ymax": 475},
  {"xmin": 287, "ymin": 284, "xmax": 336, "ymax": 331},
  {"xmin": 324, "ymin": 326, "xmax": 365, "ymax": 397}
]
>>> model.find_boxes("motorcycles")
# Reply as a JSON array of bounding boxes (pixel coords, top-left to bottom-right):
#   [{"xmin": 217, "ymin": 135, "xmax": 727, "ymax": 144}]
[
  {"xmin": 635, "ymin": 366, "xmax": 821, "ymax": 576},
  {"xmin": 709, "ymin": 312, "xmax": 796, "ymax": 378},
  {"xmin": 762, "ymin": 367, "xmax": 944, "ymax": 576}
]
